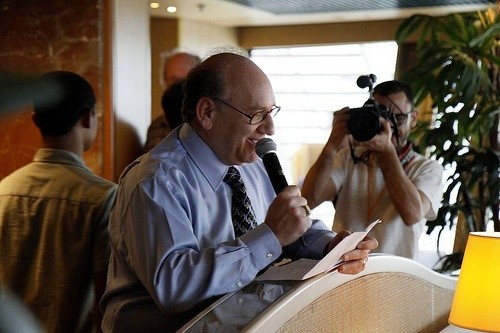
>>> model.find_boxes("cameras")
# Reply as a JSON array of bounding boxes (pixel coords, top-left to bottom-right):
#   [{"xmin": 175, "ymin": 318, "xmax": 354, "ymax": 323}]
[{"xmin": 346, "ymin": 74, "xmax": 400, "ymax": 141}]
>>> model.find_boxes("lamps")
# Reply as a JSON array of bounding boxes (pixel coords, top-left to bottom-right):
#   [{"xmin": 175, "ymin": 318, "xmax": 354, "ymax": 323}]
[{"xmin": 448, "ymin": 232, "xmax": 500, "ymax": 333}]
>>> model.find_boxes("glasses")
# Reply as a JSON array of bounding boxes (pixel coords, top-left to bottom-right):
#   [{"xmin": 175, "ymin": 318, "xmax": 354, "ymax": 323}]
[
  {"xmin": 210, "ymin": 95, "xmax": 281, "ymax": 125},
  {"xmin": 395, "ymin": 109, "xmax": 412, "ymax": 125}
]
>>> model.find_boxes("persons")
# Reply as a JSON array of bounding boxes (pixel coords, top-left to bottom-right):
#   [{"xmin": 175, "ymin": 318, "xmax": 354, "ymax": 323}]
[
  {"xmin": 300, "ymin": 79, "xmax": 443, "ymax": 262},
  {"xmin": 98, "ymin": 52, "xmax": 378, "ymax": 333},
  {"xmin": 141, "ymin": 53, "xmax": 203, "ymax": 155},
  {"xmin": 0, "ymin": 70, "xmax": 121, "ymax": 333}
]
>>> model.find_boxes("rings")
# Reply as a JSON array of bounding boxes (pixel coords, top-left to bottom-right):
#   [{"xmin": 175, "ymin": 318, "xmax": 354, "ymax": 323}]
[{"xmin": 301, "ymin": 205, "xmax": 312, "ymax": 217}]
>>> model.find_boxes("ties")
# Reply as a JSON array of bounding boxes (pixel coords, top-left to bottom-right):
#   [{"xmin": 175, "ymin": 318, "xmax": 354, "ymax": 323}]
[{"xmin": 222, "ymin": 166, "xmax": 258, "ymax": 241}]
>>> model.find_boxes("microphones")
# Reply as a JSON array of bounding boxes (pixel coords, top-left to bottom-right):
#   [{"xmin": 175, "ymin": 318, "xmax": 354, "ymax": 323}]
[{"xmin": 255, "ymin": 138, "xmax": 308, "ymax": 247}]
